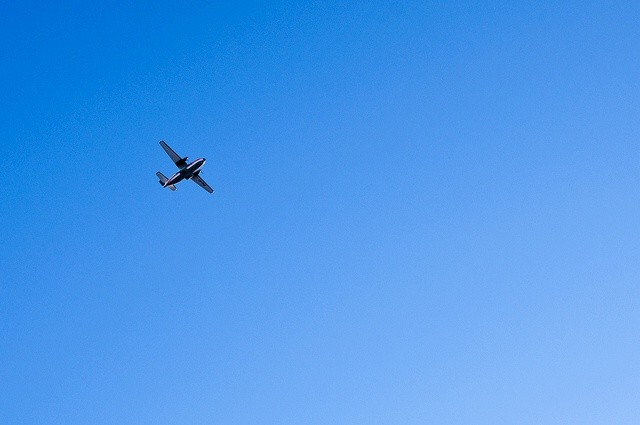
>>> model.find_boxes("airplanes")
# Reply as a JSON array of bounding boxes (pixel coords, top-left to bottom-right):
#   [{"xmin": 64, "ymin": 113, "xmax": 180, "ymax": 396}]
[{"xmin": 156, "ymin": 140, "xmax": 214, "ymax": 193}]
